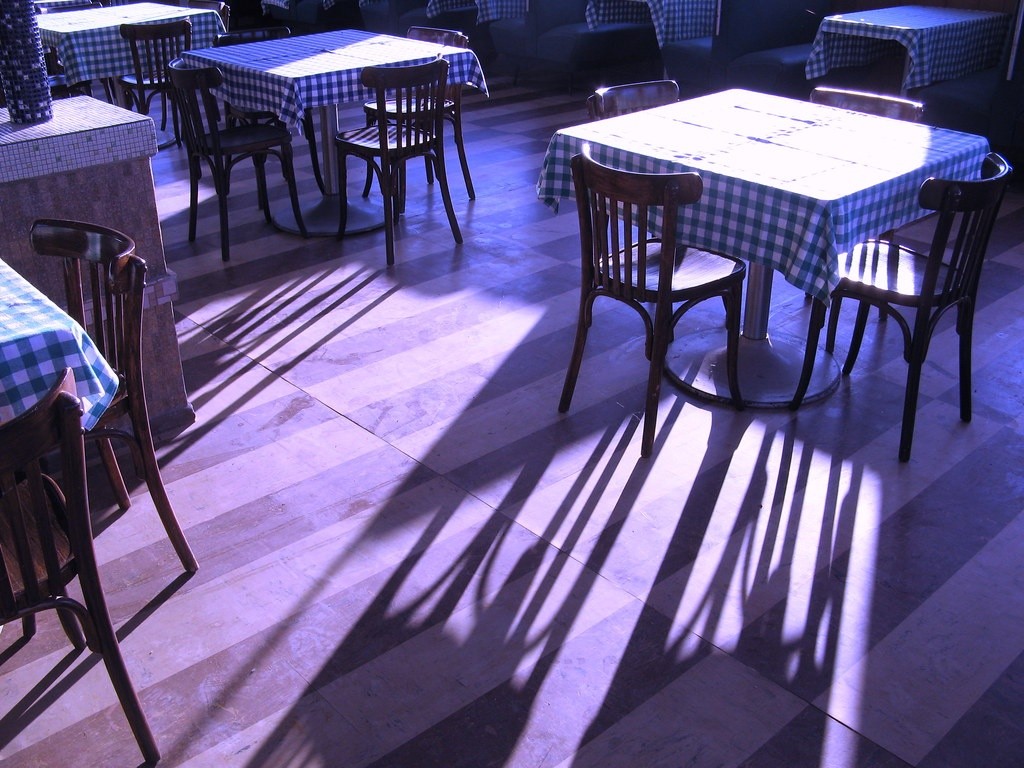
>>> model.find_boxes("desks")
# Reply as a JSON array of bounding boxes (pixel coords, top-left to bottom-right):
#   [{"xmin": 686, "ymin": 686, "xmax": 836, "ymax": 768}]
[
  {"xmin": 535, "ymin": 83, "xmax": 994, "ymax": 407},
  {"xmin": 32, "ymin": 2, "xmax": 227, "ymax": 150},
  {"xmin": 177, "ymin": 27, "xmax": 489, "ymax": 238},
  {"xmin": 0, "ymin": 253, "xmax": 117, "ymax": 611},
  {"xmin": 424, "ymin": 0, "xmax": 1014, "ymax": 121}
]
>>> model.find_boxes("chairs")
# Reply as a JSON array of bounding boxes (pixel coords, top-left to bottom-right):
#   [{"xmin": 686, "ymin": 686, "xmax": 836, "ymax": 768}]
[
  {"xmin": 186, "ymin": 0, "xmax": 293, "ymax": 163},
  {"xmin": 556, "ymin": 152, "xmax": 750, "ymax": 454},
  {"xmin": 361, "ymin": 27, "xmax": 477, "ymax": 200},
  {"xmin": 786, "ymin": 151, "xmax": 1016, "ymax": 462},
  {"xmin": 807, "ymin": 84, "xmax": 925, "ymax": 321},
  {"xmin": 167, "ymin": 25, "xmax": 330, "ymax": 261},
  {"xmin": 0, "ymin": 367, "xmax": 162, "ymax": 765},
  {"xmin": 27, "ymin": 216, "xmax": 199, "ymax": 574},
  {"xmin": 587, "ymin": 80, "xmax": 683, "ymax": 122},
  {"xmin": 113, "ymin": 17, "xmax": 193, "ymax": 148},
  {"xmin": 329, "ymin": 58, "xmax": 464, "ymax": 265}
]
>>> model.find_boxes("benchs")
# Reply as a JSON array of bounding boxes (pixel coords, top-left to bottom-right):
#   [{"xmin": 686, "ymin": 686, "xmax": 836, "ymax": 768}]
[
  {"xmin": 358, "ymin": 0, "xmax": 425, "ymax": 38},
  {"xmin": 660, "ymin": 1, "xmax": 815, "ymax": 92},
  {"xmin": 487, "ymin": 1, "xmax": 655, "ymax": 97},
  {"xmin": 920, "ymin": 62, "xmax": 1004, "ymax": 133}
]
